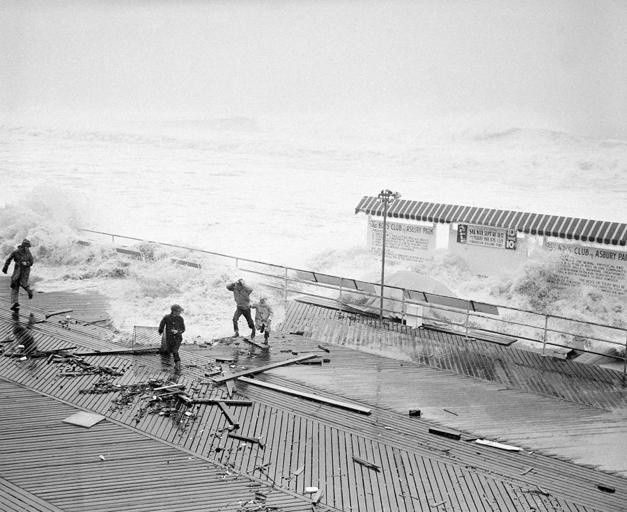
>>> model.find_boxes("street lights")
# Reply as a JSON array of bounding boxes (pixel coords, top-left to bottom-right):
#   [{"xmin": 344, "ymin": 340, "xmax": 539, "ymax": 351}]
[{"xmin": 378, "ymin": 188, "xmax": 401, "ymax": 318}]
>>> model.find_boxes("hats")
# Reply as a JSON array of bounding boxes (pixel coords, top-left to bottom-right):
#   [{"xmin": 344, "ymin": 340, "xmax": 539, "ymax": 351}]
[{"xmin": 171, "ymin": 304, "xmax": 184, "ymax": 313}]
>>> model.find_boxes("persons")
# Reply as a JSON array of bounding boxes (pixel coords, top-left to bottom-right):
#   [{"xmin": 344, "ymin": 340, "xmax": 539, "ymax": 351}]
[
  {"xmin": 245, "ymin": 295, "xmax": 272, "ymax": 346},
  {"xmin": 223, "ymin": 278, "xmax": 256, "ymax": 339},
  {"xmin": 2, "ymin": 239, "xmax": 33, "ymax": 311},
  {"xmin": 157, "ymin": 304, "xmax": 186, "ymax": 373}
]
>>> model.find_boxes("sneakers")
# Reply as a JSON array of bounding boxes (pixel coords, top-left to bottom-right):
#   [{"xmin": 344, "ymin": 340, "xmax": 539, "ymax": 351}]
[
  {"xmin": 9, "ymin": 302, "xmax": 20, "ymax": 309},
  {"xmin": 231, "ymin": 327, "xmax": 269, "ymax": 346},
  {"xmin": 28, "ymin": 289, "xmax": 34, "ymax": 299}
]
